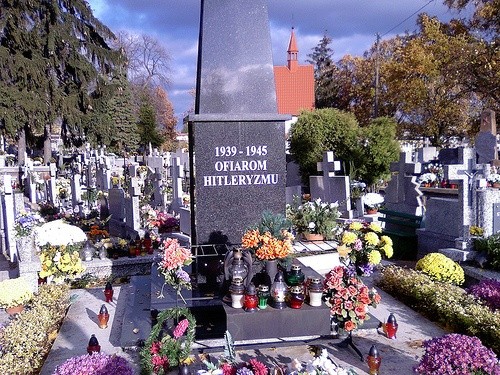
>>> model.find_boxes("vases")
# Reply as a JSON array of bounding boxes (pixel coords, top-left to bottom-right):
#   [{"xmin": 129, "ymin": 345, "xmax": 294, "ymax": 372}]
[
  {"xmin": 152, "ymin": 306, "xmax": 227, "ymax": 339},
  {"xmin": 303, "ymin": 233, "xmax": 323, "ymax": 242},
  {"xmin": 5, "ymin": 305, "xmax": 25, "ymax": 315},
  {"xmin": 263, "ymin": 258, "xmax": 280, "ymax": 283},
  {"xmin": 368, "ymin": 209, "xmax": 378, "ymax": 215}
]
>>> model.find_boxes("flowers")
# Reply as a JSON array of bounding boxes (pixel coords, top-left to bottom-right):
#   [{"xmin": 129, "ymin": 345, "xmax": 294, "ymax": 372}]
[
  {"xmin": 0, "ymin": 276, "xmax": 31, "ymax": 309},
  {"xmin": 155, "ymin": 237, "xmax": 196, "ymax": 324},
  {"xmin": 363, "ymin": 193, "xmax": 384, "ymax": 210},
  {"xmin": 285, "ymin": 193, "xmax": 344, "ymax": 241},
  {"xmin": 241, "ymin": 211, "xmax": 295, "ymax": 270},
  {"xmin": 323, "ymin": 266, "xmax": 381, "ymax": 335}
]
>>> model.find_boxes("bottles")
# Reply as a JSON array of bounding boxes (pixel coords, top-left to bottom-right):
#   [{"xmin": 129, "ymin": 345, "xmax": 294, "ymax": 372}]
[
  {"xmin": 257, "ymin": 285, "xmax": 270, "ymax": 310},
  {"xmin": 112, "ymin": 233, "xmax": 154, "ymax": 259},
  {"xmin": 99, "ymin": 247, "xmax": 106, "ymax": 259},
  {"xmin": 306, "ymin": 276, "xmax": 323, "ymax": 306},
  {"xmin": 82, "ymin": 241, "xmax": 96, "ymax": 261},
  {"xmin": 386, "ymin": 313, "xmax": 399, "ymax": 339},
  {"xmin": 244, "ymin": 282, "xmax": 259, "ymax": 312},
  {"xmin": 270, "ymin": 271, "xmax": 288, "ymax": 309},
  {"xmin": 104, "ymin": 282, "xmax": 113, "ymax": 302},
  {"xmin": 421, "ymin": 178, "xmax": 450, "ymax": 187},
  {"xmin": 87, "ymin": 334, "xmax": 100, "ymax": 356},
  {"xmin": 367, "ymin": 344, "xmax": 381, "ymax": 375},
  {"xmin": 180, "ymin": 363, "xmax": 190, "ymax": 375},
  {"xmin": 98, "ymin": 304, "xmax": 110, "ymax": 329},
  {"xmin": 287, "ymin": 266, "xmax": 305, "ymax": 308},
  {"xmin": 229, "ymin": 247, "xmax": 249, "ymax": 309}
]
